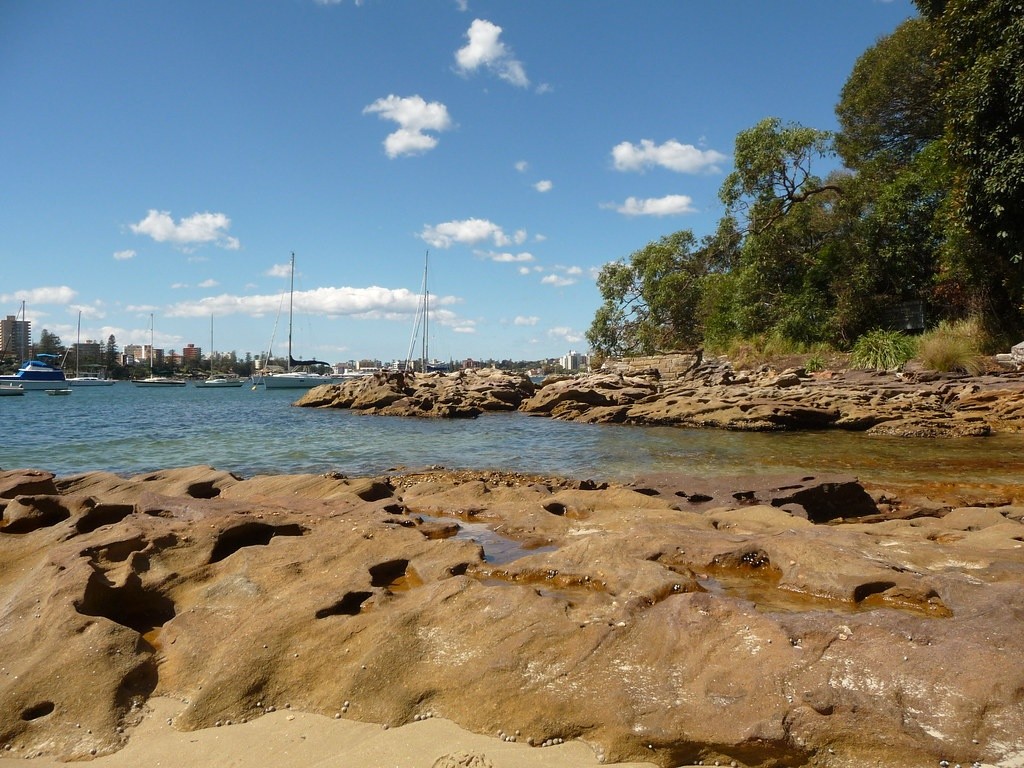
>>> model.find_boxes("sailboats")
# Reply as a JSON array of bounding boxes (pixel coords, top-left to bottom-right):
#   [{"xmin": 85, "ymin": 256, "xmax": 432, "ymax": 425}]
[
  {"xmin": 132, "ymin": 313, "xmax": 187, "ymax": 386},
  {"xmin": 59, "ymin": 310, "xmax": 119, "ymax": 386},
  {"xmin": 403, "ymin": 251, "xmax": 432, "ymax": 373},
  {"xmin": 263, "ymin": 251, "xmax": 334, "ymax": 388},
  {"xmin": 194, "ymin": 313, "xmax": 245, "ymax": 387}
]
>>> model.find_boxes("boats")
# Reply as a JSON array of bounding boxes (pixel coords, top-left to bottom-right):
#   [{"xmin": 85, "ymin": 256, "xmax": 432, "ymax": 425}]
[
  {"xmin": 45, "ymin": 389, "xmax": 73, "ymax": 396},
  {"xmin": 0, "ymin": 354, "xmax": 71, "ymax": 390},
  {"xmin": 0, "ymin": 382, "xmax": 24, "ymax": 396},
  {"xmin": 251, "ymin": 374, "xmax": 267, "ymax": 385}
]
